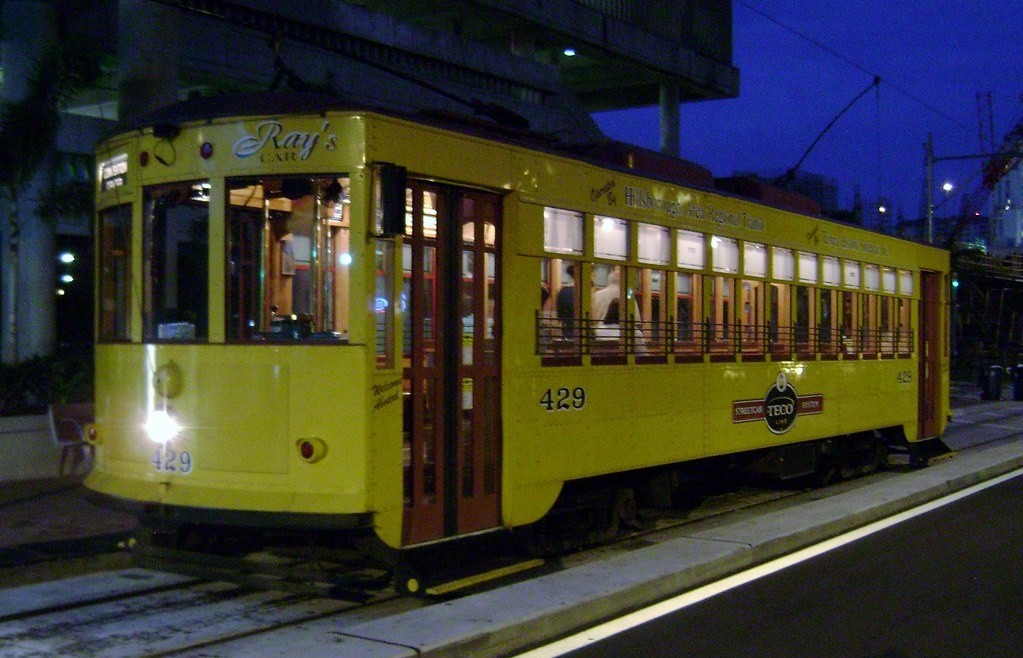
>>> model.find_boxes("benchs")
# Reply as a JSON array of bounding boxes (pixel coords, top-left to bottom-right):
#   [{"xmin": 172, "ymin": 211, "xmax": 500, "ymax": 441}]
[{"xmin": 48, "ymin": 401, "xmax": 95, "ymax": 476}]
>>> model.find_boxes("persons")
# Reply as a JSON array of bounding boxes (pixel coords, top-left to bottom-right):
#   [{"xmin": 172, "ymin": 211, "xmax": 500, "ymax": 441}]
[
  {"xmin": 555, "ymin": 266, "xmax": 575, "ymax": 339},
  {"xmin": 592, "ymin": 272, "xmax": 650, "ymax": 356}
]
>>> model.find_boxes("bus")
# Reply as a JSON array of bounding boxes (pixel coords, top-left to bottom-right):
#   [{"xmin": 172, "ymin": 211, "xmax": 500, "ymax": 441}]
[{"xmin": 82, "ymin": 98, "xmax": 954, "ymax": 562}]
